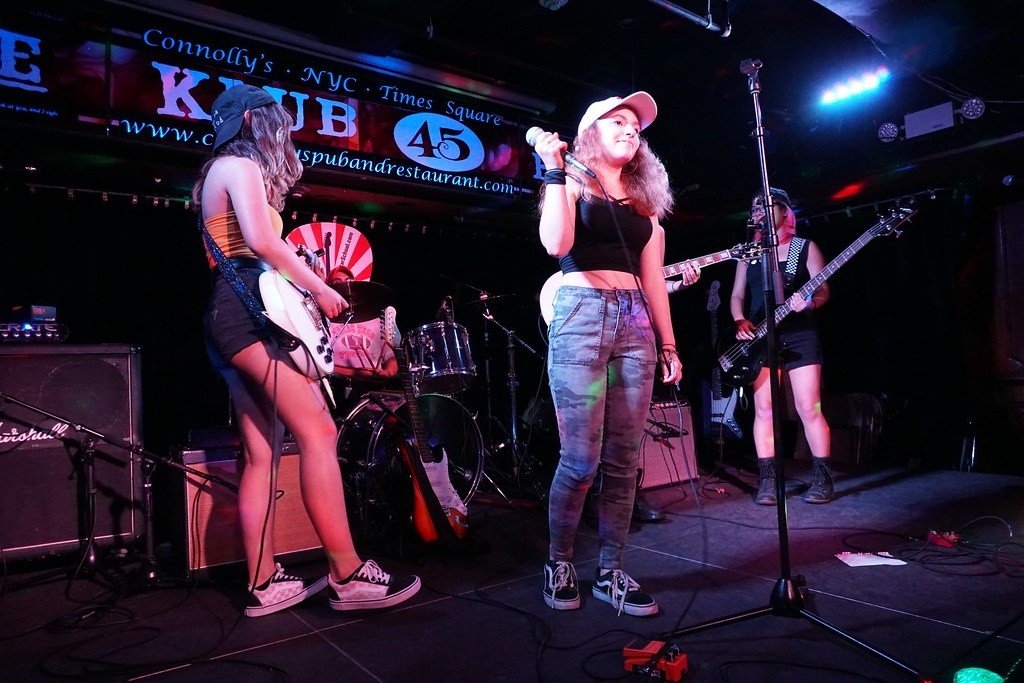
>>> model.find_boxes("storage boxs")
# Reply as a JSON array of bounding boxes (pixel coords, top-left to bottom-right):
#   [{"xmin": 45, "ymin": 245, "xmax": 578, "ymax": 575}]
[{"xmin": 161, "ymin": 432, "xmax": 324, "ymax": 570}]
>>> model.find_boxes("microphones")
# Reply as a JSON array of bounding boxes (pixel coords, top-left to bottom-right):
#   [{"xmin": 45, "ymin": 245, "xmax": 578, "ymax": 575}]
[
  {"xmin": 526, "ymin": 126, "xmax": 597, "ymax": 179},
  {"xmin": 434, "ymin": 297, "xmax": 450, "ymax": 321}
]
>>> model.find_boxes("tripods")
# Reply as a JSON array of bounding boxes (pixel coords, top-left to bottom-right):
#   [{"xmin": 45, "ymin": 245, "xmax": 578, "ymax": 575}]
[
  {"xmin": 0, "ymin": 395, "xmax": 240, "ymax": 620},
  {"xmin": 482, "ymin": 300, "xmax": 552, "ymax": 520},
  {"xmin": 651, "ymin": 62, "xmax": 929, "ymax": 683}
]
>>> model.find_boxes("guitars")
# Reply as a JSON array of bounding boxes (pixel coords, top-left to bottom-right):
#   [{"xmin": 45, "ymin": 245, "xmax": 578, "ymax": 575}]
[
  {"xmin": 537, "ymin": 242, "xmax": 766, "ymax": 328},
  {"xmin": 392, "ymin": 342, "xmax": 469, "ymax": 544},
  {"xmin": 253, "ymin": 244, "xmax": 337, "ymax": 382},
  {"xmin": 714, "ymin": 203, "xmax": 915, "ymax": 385},
  {"xmin": 697, "ymin": 278, "xmax": 745, "ymax": 438}
]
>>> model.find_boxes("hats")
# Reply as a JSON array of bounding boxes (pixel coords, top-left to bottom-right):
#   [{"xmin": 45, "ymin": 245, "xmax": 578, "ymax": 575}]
[
  {"xmin": 755, "ymin": 188, "xmax": 796, "ymax": 207},
  {"xmin": 578, "ymin": 91, "xmax": 658, "ymax": 141},
  {"xmin": 211, "ymin": 84, "xmax": 278, "ymax": 154}
]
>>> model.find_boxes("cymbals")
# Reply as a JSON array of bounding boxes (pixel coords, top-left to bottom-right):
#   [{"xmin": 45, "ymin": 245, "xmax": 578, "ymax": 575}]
[
  {"xmin": 323, "ymin": 280, "xmax": 394, "ymax": 325},
  {"xmin": 453, "ymin": 291, "xmax": 506, "ymax": 313}
]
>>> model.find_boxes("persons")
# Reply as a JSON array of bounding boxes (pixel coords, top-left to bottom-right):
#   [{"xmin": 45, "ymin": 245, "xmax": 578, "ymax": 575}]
[
  {"xmin": 729, "ymin": 189, "xmax": 832, "ymax": 505},
  {"xmin": 326, "ymin": 266, "xmax": 398, "ymax": 378},
  {"xmin": 535, "ymin": 91, "xmax": 683, "ymax": 616},
  {"xmin": 584, "ymin": 263, "xmax": 701, "ymax": 524},
  {"xmin": 194, "ymin": 84, "xmax": 420, "ymax": 617}
]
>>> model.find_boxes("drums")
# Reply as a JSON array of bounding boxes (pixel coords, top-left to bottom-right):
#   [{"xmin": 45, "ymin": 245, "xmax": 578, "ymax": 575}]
[
  {"xmin": 335, "ymin": 390, "xmax": 486, "ymax": 543},
  {"xmin": 401, "ymin": 320, "xmax": 478, "ymax": 391}
]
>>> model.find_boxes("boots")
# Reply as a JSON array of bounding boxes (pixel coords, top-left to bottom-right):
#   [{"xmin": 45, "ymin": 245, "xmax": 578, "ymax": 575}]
[
  {"xmin": 803, "ymin": 455, "xmax": 834, "ymax": 503},
  {"xmin": 756, "ymin": 458, "xmax": 778, "ymax": 506},
  {"xmin": 581, "ymin": 462, "xmax": 600, "ymax": 530},
  {"xmin": 632, "ymin": 468, "xmax": 667, "ymax": 522}
]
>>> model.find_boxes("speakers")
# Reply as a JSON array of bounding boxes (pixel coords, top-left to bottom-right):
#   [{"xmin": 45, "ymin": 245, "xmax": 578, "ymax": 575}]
[
  {"xmin": 167, "ymin": 434, "xmax": 326, "ymax": 579},
  {"xmin": 0, "ymin": 346, "xmax": 146, "ymax": 572}
]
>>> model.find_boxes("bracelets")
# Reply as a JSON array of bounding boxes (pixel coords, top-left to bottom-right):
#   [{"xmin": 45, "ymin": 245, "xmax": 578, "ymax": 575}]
[
  {"xmin": 662, "ymin": 349, "xmax": 679, "ymax": 362},
  {"xmin": 672, "ymin": 281, "xmax": 677, "ymax": 292},
  {"xmin": 662, "ymin": 344, "xmax": 676, "ymax": 348},
  {"xmin": 544, "ymin": 167, "xmax": 567, "ymax": 184},
  {"xmin": 812, "ymin": 300, "xmax": 816, "ymax": 309}
]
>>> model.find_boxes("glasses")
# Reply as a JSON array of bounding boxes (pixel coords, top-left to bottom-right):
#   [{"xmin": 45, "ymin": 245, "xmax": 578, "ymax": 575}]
[{"xmin": 747, "ymin": 202, "xmax": 777, "ymax": 217}]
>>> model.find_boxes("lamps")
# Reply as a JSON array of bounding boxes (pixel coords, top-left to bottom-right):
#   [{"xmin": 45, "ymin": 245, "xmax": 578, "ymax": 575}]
[{"xmin": 878, "ymin": 97, "xmax": 986, "ymax": 147}]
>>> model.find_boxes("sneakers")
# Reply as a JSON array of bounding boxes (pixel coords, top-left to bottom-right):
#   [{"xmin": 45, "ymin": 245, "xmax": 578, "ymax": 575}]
[
  {"xmin": 327, "ymin": 559, "xmax": 422, "ymax": 610},
  {"xmin": 242, "ymin": 563, "xmax": 328, "ymax": 616},
  {"xmin": 592, "ymin": 567, "xmax": 659, "ymax": 617},
  {"xmin": 542, "ymin": 559, "xmax": 581, "ymax": 610}
]
